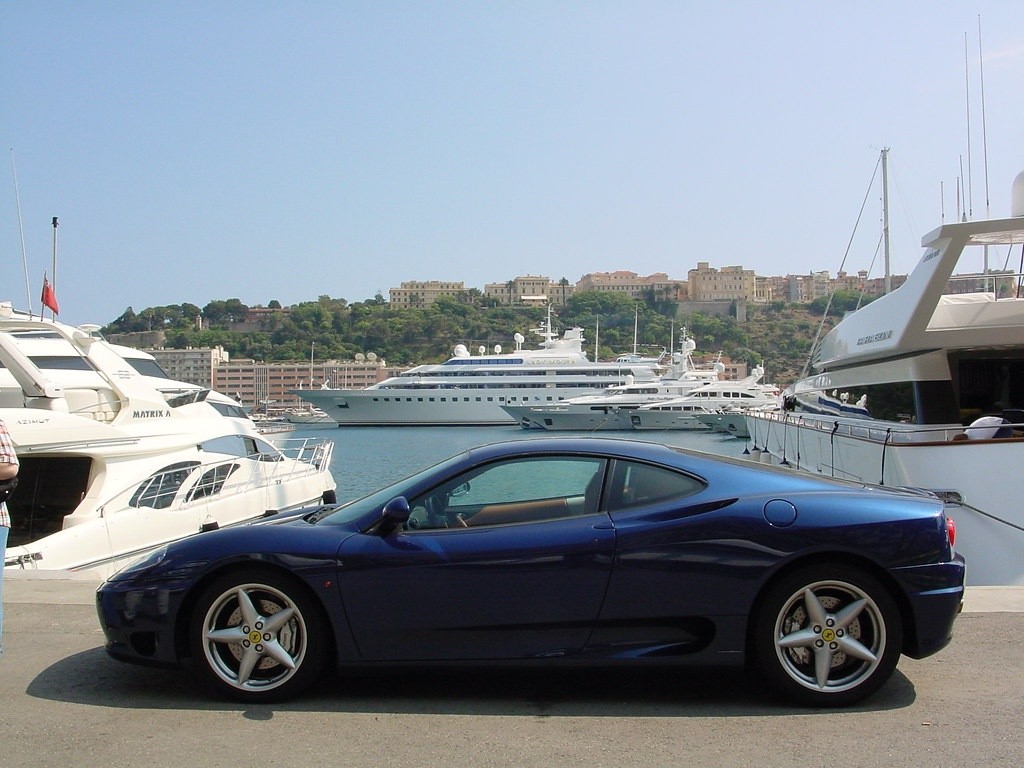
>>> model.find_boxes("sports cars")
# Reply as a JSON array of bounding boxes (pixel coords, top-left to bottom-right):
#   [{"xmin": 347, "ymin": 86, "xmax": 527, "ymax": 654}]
[{"xmin": 91, "ymin": 436, "xmax": 965, "ymax": 709}]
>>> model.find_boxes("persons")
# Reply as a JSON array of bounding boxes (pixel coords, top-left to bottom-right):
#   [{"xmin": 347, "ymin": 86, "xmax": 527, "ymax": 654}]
[{"xmin": 0, "ymin": 410, "xmax": 19, "ymax": 655}]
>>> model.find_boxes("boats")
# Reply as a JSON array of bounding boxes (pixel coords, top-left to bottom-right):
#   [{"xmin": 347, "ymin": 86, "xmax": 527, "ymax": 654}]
[
  {"xmin": 742, "ymin": 12, "xmax": 1023, "ymax": 586},
  {"xmin": 0, "ymin": 145, "xmax": 786, "ymax": 583}
]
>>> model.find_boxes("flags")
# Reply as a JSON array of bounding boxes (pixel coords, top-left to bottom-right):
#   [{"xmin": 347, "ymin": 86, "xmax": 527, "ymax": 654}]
[{"xmin": 41, "ymin": 269, "xmax": 58, "ymax": 316}]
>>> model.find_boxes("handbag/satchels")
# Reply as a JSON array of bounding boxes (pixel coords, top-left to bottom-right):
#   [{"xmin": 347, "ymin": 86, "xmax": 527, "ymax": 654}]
[{"xmin": 0, "ymin": 476, "xmax": 18, "ymax": 503}]
